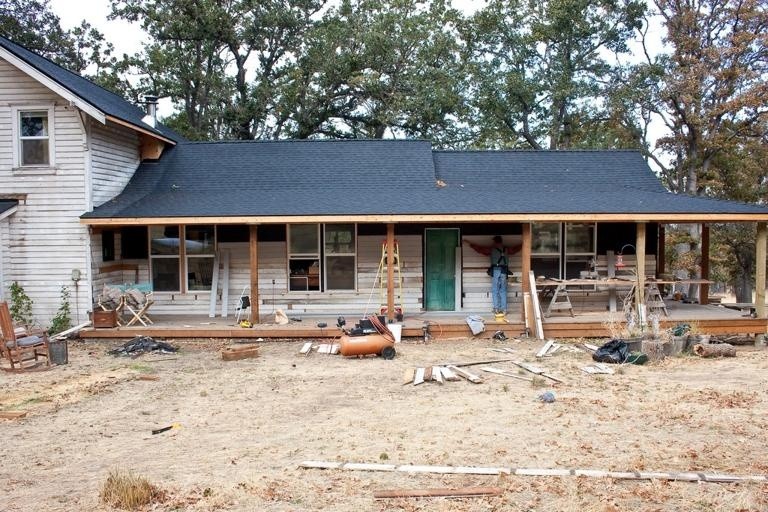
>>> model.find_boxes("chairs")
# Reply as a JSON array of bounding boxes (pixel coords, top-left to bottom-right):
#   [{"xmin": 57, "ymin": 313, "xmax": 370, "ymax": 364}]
[
  {"xmin": 98, "ymin": 282, "xmax": 155, "ymax": 329},
  {"xmin": 0, "ymin": 301, "xmax": 56, "ymax": 374}
]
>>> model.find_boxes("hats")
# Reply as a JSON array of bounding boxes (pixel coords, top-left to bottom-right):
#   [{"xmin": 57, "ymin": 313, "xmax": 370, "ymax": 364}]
[{"xmin": 492, "ymin": 236, "xmax": 503, "ymax": 243}]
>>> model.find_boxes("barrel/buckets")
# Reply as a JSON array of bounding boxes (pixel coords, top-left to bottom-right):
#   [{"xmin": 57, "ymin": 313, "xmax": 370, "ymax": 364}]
[
  {"xmin": 47, "ymin": 341, "xmax": 68, "ymax": 363},
  {"xmin": 385, "ymin": 323, "xmax": 402, "ymax": 342},
  {"xmin": 495, "ymin": 314, "xmax": 504, "ymax": 322}
]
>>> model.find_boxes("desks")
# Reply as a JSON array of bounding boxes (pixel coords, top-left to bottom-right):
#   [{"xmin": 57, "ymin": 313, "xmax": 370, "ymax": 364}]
[{"xmin": 537, "ymin": 278, "xmax": 676, "ymax": 319}]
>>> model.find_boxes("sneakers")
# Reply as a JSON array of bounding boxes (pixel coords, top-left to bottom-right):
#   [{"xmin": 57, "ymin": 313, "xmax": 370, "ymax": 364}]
[{"xmin": 492, "ymin": 308, "xmax": 507, "ymax": 314}]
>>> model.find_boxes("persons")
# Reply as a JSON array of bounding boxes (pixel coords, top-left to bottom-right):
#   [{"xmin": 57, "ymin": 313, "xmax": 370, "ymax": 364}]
[{"xmin": 462, "ymin": 236, "xmax": 522, "ymax": 314}]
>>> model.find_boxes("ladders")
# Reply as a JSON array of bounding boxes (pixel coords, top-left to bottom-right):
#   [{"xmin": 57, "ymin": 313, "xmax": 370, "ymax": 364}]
[{"xmin": 380, "ymin": 239, "xmax": 404, "ymax": 322}]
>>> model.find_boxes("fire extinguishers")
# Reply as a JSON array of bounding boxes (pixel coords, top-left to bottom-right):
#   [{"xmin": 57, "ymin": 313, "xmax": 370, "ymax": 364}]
[{"xmin": 616, "ymin": 244, "xmax": 636, "ymax": 270}]
